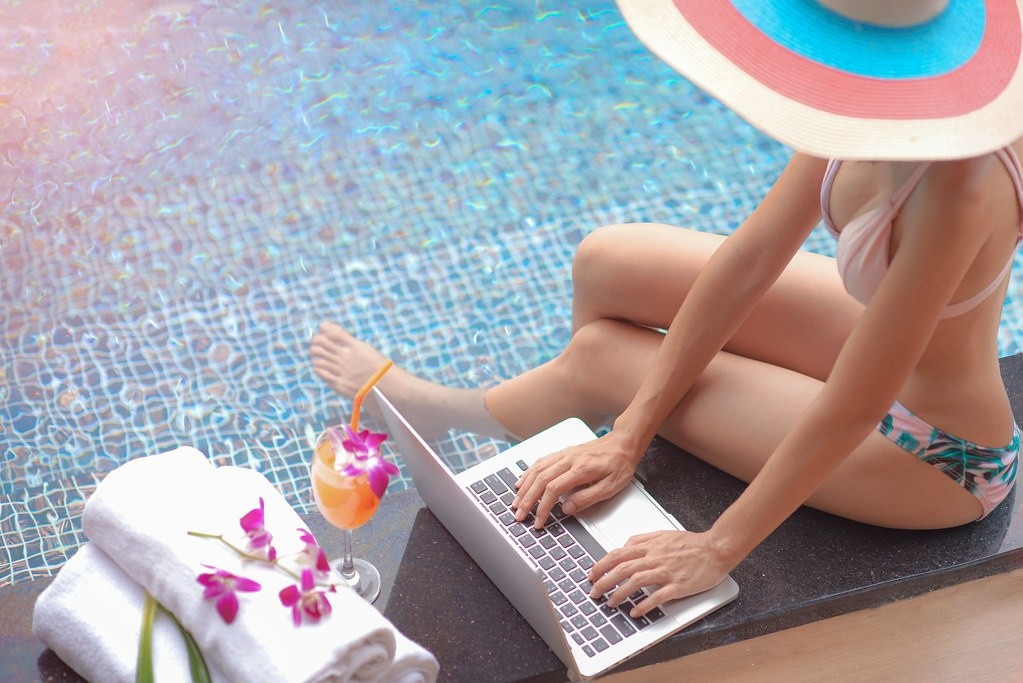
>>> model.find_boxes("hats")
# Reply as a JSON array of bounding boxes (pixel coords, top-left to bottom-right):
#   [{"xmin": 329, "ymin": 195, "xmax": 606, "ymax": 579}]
[{"xmin": 615, "ymin": 0, "xmax": 1023, "ymax": 161}]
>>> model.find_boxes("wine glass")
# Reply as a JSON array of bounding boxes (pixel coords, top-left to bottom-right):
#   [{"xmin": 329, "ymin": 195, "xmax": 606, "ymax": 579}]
[{"xmin": 310, "ymin": 422, "xmax": 383, "ymax": 605}]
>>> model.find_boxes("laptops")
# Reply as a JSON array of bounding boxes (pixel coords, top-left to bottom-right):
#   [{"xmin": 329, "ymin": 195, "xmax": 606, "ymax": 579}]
[{"xmin": 370, "ymin": 385, "xmax": 740, "ymax": 683}]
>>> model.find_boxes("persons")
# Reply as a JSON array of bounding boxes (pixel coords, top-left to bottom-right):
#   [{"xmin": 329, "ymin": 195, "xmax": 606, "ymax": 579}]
[{"xmin": 309, "ymin": 1, "xmax": 1023, "ymax": 618}]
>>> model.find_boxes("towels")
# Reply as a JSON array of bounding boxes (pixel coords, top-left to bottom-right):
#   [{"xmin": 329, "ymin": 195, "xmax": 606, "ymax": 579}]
[{"xmin": 31, "ymin": 445, "xmax": 440, "ymax": 683}]
